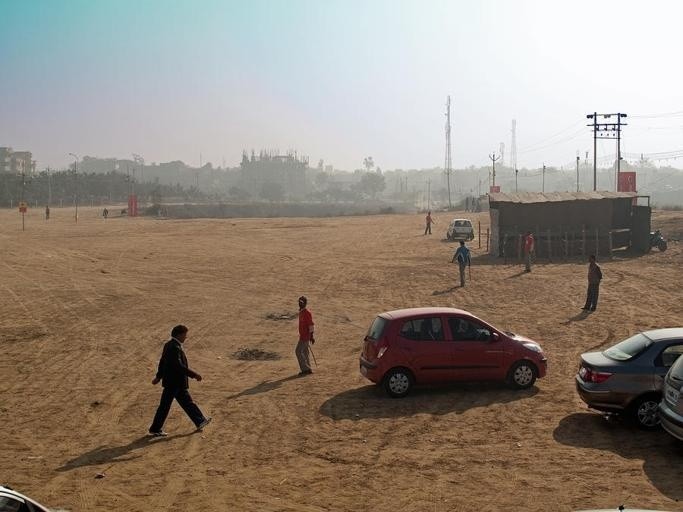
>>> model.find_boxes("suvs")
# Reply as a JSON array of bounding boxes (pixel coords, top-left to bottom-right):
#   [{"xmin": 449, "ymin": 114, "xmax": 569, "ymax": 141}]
[{"xmin": 447, "ymin": 219, "xmax": 475, "ymax": 241}]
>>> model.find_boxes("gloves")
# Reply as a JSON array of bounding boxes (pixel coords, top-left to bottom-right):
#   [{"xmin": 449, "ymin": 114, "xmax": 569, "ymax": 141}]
[{"xmin": 310, "ymin": 338, "xmax": 315, "ymax": 344}]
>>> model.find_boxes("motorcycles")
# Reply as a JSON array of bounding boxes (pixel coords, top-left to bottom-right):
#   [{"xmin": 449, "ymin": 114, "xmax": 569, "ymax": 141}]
[{"xmin": 650, "ymin": 229, "xmax": 667, "ymax": 251}]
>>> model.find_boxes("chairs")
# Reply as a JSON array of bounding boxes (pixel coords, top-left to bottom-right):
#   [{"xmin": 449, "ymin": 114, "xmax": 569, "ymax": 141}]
[
  {"xmin": 436, "ymin": 318, "xmax": 460, "ymax": 341},
  {"xmin": 421, "ymin": 318, "xmax": 436, "ymax": 341}
]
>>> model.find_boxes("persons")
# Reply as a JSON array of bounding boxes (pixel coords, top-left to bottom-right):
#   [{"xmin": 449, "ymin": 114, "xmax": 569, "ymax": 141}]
[
  {"xmin": 524, "ymin": 231, "xmax": 534, "ymax": 272},
  {"xmin": 295, "ymin": 295, "xmax": 315, "ymax": 375},
  {"xmin": 465, "ymin": 198, "xmax": 482, "ymax": 212},
  {"xmin": 425, "ymin": 212, "xmax": 435, "ymax": 235},
  {"xmin": 581, "ymin": 255, "xmax": 603, "ymax": 311},
  {"xmin": 103, "ymin": 208, "xmax": 108, "ymax": 218},
  {"xmin": 148, "ymin": 325, "xmax": 212, "ymax": 436},
  {"xmin": 46, "ymin": 205, "xmax": 50, "ymax": 220},
  {"xmin": 450, "ymin": 240, "xmax": 471, "ymax": 287}
]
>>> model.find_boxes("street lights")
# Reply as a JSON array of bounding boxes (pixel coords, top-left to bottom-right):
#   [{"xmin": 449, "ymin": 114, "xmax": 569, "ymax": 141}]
[{"xmin": 69, "ymin": 152, "xmax": 81, "ymax": 223}]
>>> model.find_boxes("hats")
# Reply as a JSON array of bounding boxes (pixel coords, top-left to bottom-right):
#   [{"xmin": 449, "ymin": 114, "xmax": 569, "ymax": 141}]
[{"xmin": 299, "ymin": 297, "xmax": 306, "ymax": 304}]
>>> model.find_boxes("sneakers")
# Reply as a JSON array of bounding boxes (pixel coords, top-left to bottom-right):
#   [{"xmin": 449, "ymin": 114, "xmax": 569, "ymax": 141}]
[
  {"xmin": 299, "ymin": 369, "xmax": 312, "ymax": 375},
  {"xmin": 149, "ymin": 430, "xmax": 168, "ymax": 436},
  {"xmin": 197, "ymin": 418, "xmax": 212, "ymax": 430}
]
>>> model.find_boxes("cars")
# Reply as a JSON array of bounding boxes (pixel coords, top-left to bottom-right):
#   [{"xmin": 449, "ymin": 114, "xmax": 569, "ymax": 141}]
[
  {"xmin": 359, "ymin": 307, "xmax": 547, "ymax": 398},
  {"xmin": 576, "ymin": 327, "xmax": 683, "ymax": 441}
]
